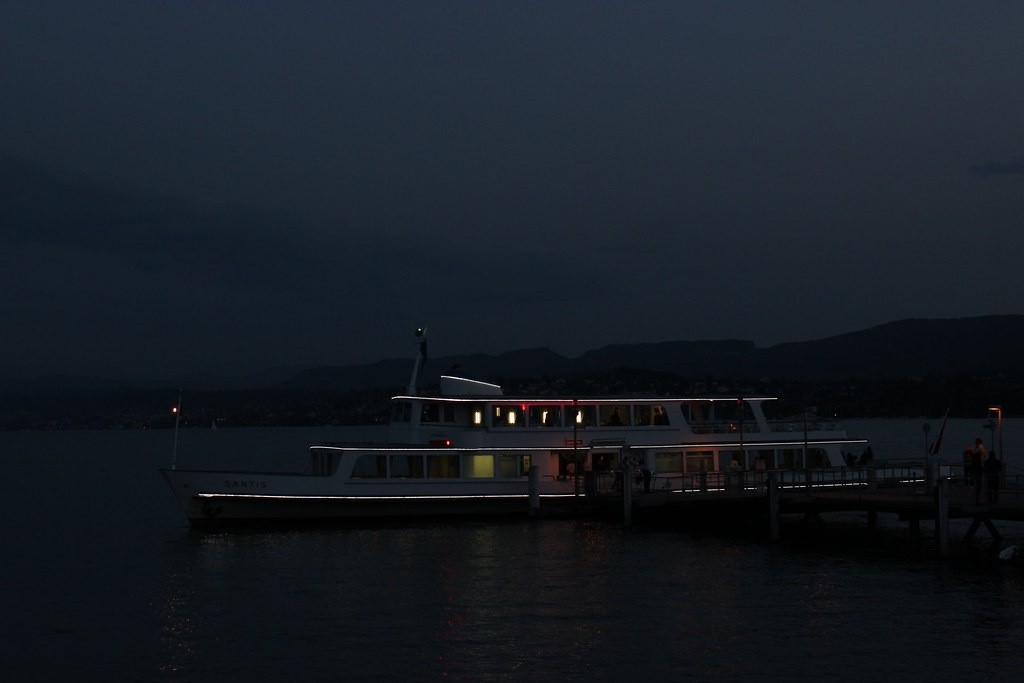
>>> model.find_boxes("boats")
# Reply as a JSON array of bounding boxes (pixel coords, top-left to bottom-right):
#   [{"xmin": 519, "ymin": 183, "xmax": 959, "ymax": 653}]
[{"xmin": 147, "ymin": 325, "xmax": 975, "ymax": 535}]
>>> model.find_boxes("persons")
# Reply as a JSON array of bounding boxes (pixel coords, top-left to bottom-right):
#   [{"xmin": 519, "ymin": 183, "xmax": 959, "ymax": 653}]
[
  {"xmin": 973, "ymin": 437, "xmax": 1002, "ymax": 505},
  {"xmin": 814, "ymin": 449, "xmax": 829, "ymax": 468},
  {"xmin": 639, "ymin": 467, "xmax": 651, "ymax": 492},
  {"xmin": 699, "ymin": 458, "xmax": 708, "ymax": 496},
  {"xmin": 847, "ymin": 446, "xmax": 874, "ymax": 465},
  {"xmin": 611, "ymin": 406, "xmax": 623, "ymax": 425}
]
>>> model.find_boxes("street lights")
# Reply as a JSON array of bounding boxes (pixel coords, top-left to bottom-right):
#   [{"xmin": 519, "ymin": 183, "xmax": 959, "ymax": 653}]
[
  {"xmin": 172, "ymin": 407, "xmax": 181, "ymax": 456},
  {"xmin": 989, "ymin": 406, "xmax": 1004, "ymax": 463}
]
